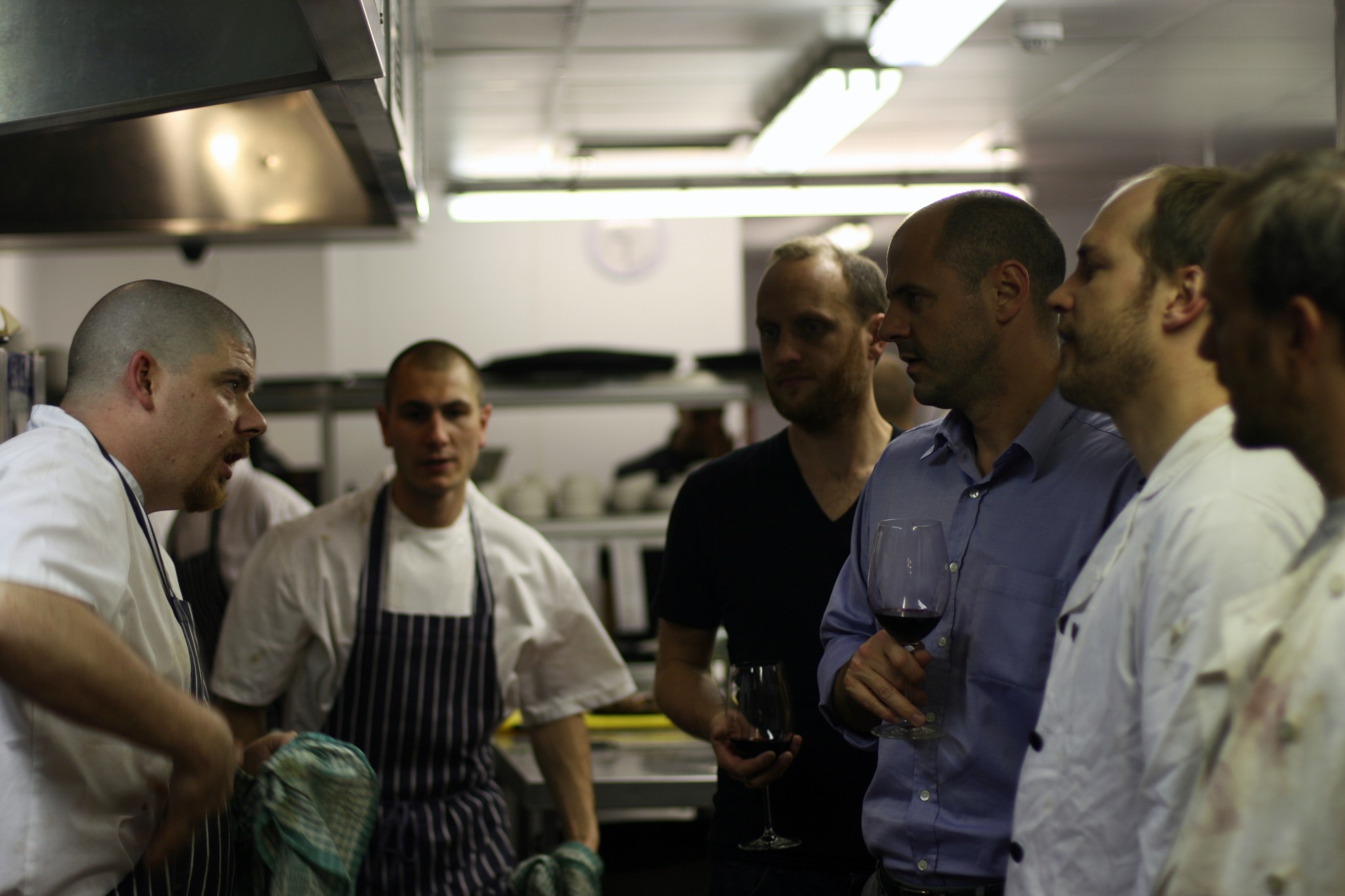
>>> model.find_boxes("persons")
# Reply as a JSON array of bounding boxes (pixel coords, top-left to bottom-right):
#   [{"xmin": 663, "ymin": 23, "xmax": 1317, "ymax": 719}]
[
  {"xmin": 1003, "ymin": 164, "xmax": 1326, "ymax": 896},
  {"xmin": 0, "ymin": 281, "xmax": 300, "ymax": 896},
  {"xmin": 206, "ymin": 340, "xmax": 638, "ymax": 896},
  {"xmin": 652, "ymin": 236, "xmax": 904, "ymax": 896},
  {"xmin": 1154, "ymin": 148, "xmax": 1345, "ymax": 896},
  {"xmin": 871, "ymin": 353, "xmax": 923, "ymax": 430},
  {"xmin": 147, "ymin": 455, "xmax": 316, "ymax": 687},
  {"xmin": 818, "ymin": 190, "xmax": 1144, "ymax": 896}
]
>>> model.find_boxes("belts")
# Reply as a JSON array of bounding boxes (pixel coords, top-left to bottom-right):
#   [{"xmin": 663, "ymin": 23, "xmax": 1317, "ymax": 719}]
[{"xmin": 878, "ymin": 861, "xmax": 1005, "ymax": 896}]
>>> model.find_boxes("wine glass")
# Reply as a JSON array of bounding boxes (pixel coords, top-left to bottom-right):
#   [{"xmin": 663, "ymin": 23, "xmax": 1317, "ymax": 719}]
[
  {"xmin": 726, "ymin": 658, "xmax": 803, "ymax": 851},
  {"xmin": 866, "ymin": 517, "xmax": 951, "ymax": 744}
]
